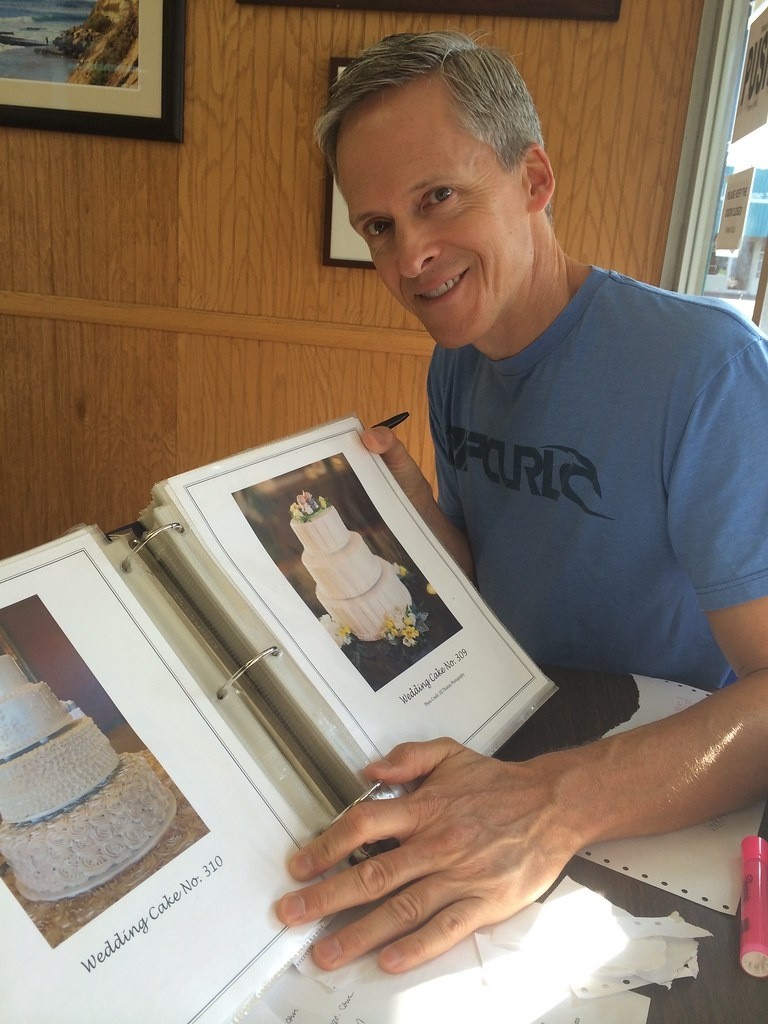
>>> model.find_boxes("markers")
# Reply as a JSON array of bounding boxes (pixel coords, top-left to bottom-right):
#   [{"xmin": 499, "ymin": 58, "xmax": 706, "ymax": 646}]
[{"xmin": 739, "ymin": 834, "xmax": 768, "ymax": 979}]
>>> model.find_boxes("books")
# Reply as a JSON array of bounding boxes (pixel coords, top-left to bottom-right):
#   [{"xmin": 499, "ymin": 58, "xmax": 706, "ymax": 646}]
[{"xmin": 0, "ymin": 417, "xmax": 559, "ymax": 1023}]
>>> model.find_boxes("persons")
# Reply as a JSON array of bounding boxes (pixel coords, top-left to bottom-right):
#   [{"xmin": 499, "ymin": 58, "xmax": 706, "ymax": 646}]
[{"xmin": 275, "ymin": 33, "xmax": 768, "ymax": 973}]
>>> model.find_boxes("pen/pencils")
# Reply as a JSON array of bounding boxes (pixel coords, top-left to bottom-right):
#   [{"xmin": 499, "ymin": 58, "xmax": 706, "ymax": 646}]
[{"xmin": 371, "ymin": 412, "xmax": 409, "ymax": 429}]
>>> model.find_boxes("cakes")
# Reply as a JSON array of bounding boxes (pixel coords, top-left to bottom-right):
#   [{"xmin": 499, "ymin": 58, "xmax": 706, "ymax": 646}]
[
  {"xmin": 289, "ymin": 490, "xmax": 411, "ymax": 641},
  {"xmin": 0, "ymin": 653, "xmax": 174, "ymax": 891}
]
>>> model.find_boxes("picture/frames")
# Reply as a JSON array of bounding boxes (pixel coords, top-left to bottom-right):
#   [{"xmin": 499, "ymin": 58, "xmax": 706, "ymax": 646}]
[
  {"xmin": 0, "ymin": 0, "xmax": 187, "ymax": 144},
  {"xmin": 322, "ymin": 56, "xmax": 375, "ymax": 271}
]
